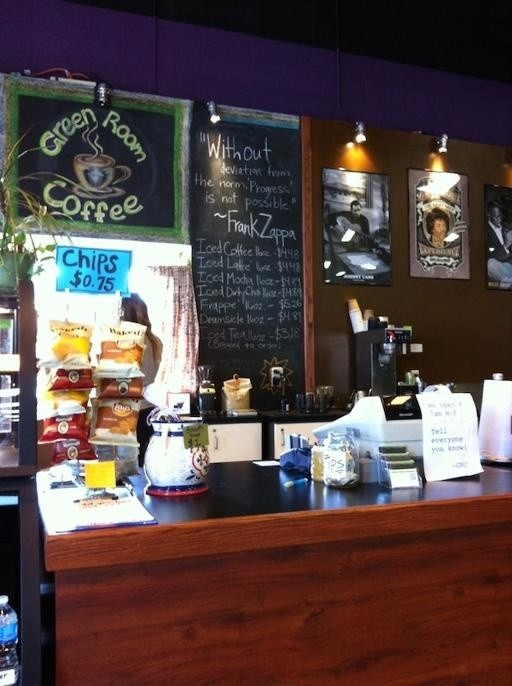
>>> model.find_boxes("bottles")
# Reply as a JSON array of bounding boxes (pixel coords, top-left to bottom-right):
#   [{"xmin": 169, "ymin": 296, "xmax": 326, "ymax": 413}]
[
  {"xmin": 322, "ymin": 431, "xmax": 362, "ymax": 490},
  {"xmin": 348, "ymin": 298, "xmax": 365, "ymax": 334},
  {"xmin": 0, "ymin": 596, "xmax": 21, "ymax": 686},
  {"xmin": 278, "ymin": 374, "xmax": 293, "ymax": 412},
  {"xmin": 415, "ymin": 375, "xmax": 423, "ymax": 392}
]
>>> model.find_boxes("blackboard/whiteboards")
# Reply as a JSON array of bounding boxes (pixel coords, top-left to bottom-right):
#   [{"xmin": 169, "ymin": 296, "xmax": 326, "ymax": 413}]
[{"xmin": 185, "ymin": 100, "xmax": 315, "ymax": 412}]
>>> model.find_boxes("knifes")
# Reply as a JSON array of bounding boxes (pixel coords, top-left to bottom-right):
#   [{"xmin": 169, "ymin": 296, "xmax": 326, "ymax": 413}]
[{"xmin": 197, "ymin": 365, "xmax": 212, "ymax": 382}]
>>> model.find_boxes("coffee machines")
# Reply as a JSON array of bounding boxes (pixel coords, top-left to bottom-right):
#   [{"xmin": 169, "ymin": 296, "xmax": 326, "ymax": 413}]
[{"xmin": 353, "ymin": 328, "xmax": 414, "ymax": 421}]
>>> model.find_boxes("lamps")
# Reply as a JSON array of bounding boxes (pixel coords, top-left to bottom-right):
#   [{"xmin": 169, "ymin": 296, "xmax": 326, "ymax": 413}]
[
  {"xmin": 429, "ymin": 126, "xmax": 456, "ymax": 157},
  {"xmin": 343, "ymin": 116, "xmax": 372, "ymax": 153},
  {"xmin": 94, "ymin": 82, "xmax": 112, "ymax": 106},
  {"xmin": 203, "ymin": 99, "xmax": 222, "ymax": 125}
]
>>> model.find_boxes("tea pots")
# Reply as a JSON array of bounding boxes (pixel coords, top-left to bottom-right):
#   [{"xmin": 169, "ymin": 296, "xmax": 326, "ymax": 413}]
[{"xmin": 142, "ymin": 414, "xmax": 210, "ymax": 491}]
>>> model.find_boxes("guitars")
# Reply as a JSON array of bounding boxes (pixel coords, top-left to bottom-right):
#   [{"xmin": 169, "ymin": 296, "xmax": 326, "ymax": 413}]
[{"xmin": 323, "ymin": 216, "xmax": 383, "ymax": 249}]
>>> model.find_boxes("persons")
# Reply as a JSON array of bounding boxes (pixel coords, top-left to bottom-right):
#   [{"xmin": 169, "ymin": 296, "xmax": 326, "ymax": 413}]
[
  {"xmin": 426, "ymin": 208, "xmax": 450, "ymax": 247},
  {"xmin": 325, "ymin": 199, "xmax": 369, "ymax": 249},
  {"xmin": 486, "ymin": 199, "xmax": 512, "ymax": 260}
]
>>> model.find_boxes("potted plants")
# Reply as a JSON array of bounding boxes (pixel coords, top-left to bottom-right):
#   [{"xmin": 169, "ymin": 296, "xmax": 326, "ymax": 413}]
[{"xmin": 0, "ymin": 121, "xmax": 92, "ymax": 300}]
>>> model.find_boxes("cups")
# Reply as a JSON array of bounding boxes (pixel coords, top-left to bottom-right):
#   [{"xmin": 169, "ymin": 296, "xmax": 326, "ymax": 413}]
[
  {"xmin": 368, "ymin": 318, "xmax": 381, "ymax": 331},
  {"xmin": 362, "ymin": 308, "xmax": 377, "ymax": 330},
  {"xmin": 72, "ymin": 152, "xmax": 130, "ymax": 192},
  {"xmin": 295, "ymin": 390, "xmax": 316, "ymax": 413},
  {"xmin": 317, "ymin": 385, "xmax": 335, "ymax": 412}
]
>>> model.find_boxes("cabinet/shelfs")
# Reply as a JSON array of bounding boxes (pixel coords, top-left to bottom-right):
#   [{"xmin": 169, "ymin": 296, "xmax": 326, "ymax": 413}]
[
  {"xmin": 203, "ymin": 415, "xmax": 262, "ymax": 462},
  {"xmin": 267, "ymin": 413, "xmax": 347, "ymax": 461},
  {"xmin": 0, "ymin": 273, "xmax": 45, "ymax": 686}
]
